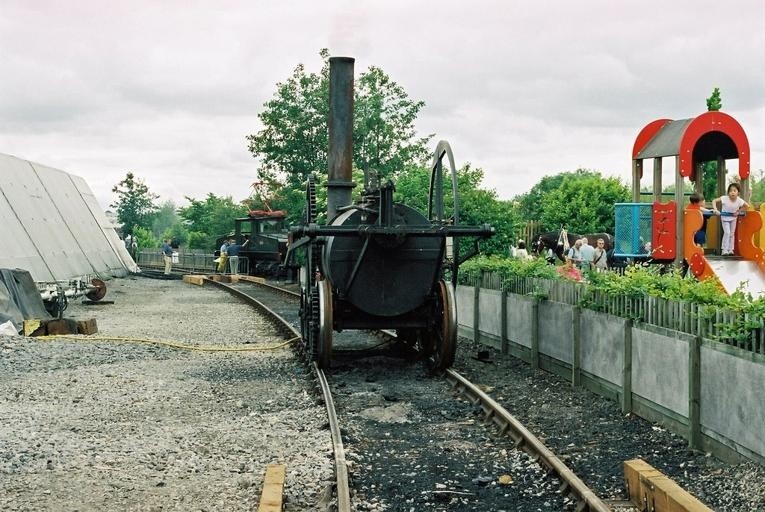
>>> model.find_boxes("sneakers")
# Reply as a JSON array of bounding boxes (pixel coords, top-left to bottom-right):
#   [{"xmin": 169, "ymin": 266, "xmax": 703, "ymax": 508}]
[{"xmin": 721, "ymin": 250, "xmax": 734, "ymax": 256}]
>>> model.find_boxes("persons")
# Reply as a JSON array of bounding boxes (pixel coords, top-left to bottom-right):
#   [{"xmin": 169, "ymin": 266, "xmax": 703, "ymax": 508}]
[
  {"xmin": 511, "ymin": 236, "xmax": 651, "ymax": 273},
  {"xmin": 120, "ymin": 233, "xmax": 138, "ymax": 255},
  {"xmin": 215, "ymin": 239, "xmax": 250, "ymax": 274},
  {"xmin": 713, "ymin": 183, "xmax": 750, "ymax": 257},
  {"xmin": 687, "ymin": 192, "xmax": 715, "ymax": 247},
  {"xmin": 163, "ymin": 240, "xmax": 173, "ymax": 274}
]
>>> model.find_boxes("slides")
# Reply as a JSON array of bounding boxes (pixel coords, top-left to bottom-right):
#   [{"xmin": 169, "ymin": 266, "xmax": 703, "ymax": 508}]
[{"xmin": 688, "ymin": 255, "xmax": 764, "ymax": 299}]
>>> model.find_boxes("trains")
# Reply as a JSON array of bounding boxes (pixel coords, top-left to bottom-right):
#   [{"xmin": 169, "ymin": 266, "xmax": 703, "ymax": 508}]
[
  {"xmin": 217, "ymin": 209, "xmax": 289, "ymax": 280},
  {"xmin": 290, "ymin": 57, "xmax": 495, "ymax": 369}
]
